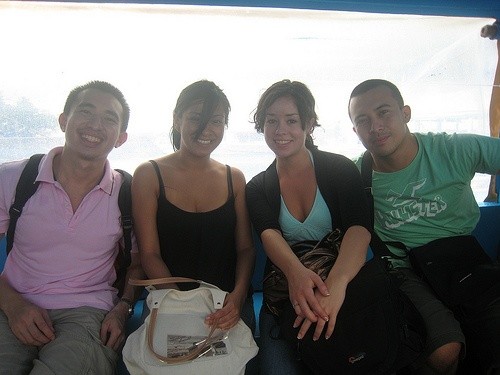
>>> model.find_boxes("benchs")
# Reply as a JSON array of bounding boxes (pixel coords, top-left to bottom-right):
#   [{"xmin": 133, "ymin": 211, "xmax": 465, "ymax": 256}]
[{"xmin": 0, "ymin": 200, "xmax": 500, "ymax": 341}]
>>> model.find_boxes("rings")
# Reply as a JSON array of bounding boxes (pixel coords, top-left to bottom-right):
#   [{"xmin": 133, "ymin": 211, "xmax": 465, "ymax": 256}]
[{"xmin": 293, "ymin": 303, "xmax": 299, "ymax": 307}]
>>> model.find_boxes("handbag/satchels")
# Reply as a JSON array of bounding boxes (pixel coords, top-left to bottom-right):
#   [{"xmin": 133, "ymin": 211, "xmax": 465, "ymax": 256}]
[
  {"xmin": 262, "ymin": 228, "xmax": 345, "ymax": 319},
  {"xmin": 121, "ymin": 276, "xmax": 259, "ymax": 375},
  {"xmin": 409, "ymin": 236, "xmax": 500, "ymax": 323}
]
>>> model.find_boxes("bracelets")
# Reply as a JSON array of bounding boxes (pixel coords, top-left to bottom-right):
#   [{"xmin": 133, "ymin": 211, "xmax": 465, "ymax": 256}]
[{"xmin": 121, "ymin": 298, "xmax": 134, "ymax": 316}]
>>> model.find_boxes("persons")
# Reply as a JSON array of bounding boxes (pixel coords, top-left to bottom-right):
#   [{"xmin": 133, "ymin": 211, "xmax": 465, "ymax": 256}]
[
  {"xmin": 349, "ymin": 79, "xmax": 500, "ymax": 375},
  {"xmin": 0, "ymin": 80, "xmax": 148, "ymax": 374},
  {"xmin": 245, "ymin": 79, "xmax": 426, "ymax": 375},
  {"xmin": 131, "ymin": 79, "xmax": 256, "ymax": 374}
]
